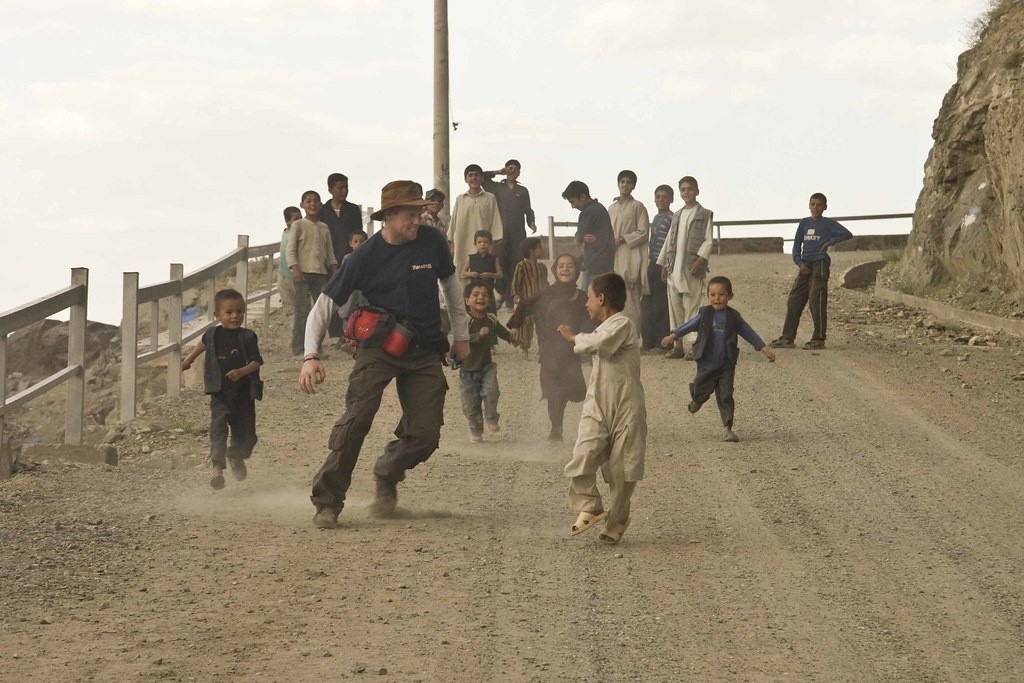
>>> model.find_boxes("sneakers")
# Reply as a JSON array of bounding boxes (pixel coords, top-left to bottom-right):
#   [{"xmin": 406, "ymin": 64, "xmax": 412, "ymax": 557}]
[
  {"xmin": 370, "ymin": 490, "xmax": 396, "ymax": 520},
  {"xmin": 313, "ymin": 499, "xmax": 342, "ymax": 528}
]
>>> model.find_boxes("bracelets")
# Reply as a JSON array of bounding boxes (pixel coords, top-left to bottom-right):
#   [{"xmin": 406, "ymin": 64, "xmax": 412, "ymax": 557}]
[
  {"xmin": 303, "ymin": 356, "xmax": 320, "ymax": 363},
  {"xmin": 619, "ymin": 236, "xmax": 623, "ymax": 243}
]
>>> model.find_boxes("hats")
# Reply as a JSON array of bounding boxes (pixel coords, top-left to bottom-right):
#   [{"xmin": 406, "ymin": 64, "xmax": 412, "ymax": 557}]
[
  {"xmin": 425, "ymin": 188, "xmax": 445, "ymax": 202},
  {"xmin": 370, "ymin": 180, "xmax": 439, "ymax": 221}
]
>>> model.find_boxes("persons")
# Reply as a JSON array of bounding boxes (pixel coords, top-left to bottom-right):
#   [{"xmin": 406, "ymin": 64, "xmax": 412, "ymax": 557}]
[
  {"xmin": 608, "ymin": 170, "xmax": 649, "ymax": 338},
  {"xmin": 767, "ymin": 193, "xmax": 853, "ymax": 349},
  {"xmin": 462, "ymin": 230, "xmax": 502, "ymax": 356},
  {"xmin": 606, "ymin": 170, "xmax": 649, "ymax": 346},
  {"xmin": 638, "ymin": 185, "xmax": 678, "ymax": 352},
  {"xmin": 481, "ymin": 159, "xmax": 537, "ymax": 312},
  {"xmin": 661, "ymin": 276, "xmax": 776, "ymax": 442},
  {"xmin": 447, "ymin": 164, "xmax": 504, "ymax": 291},
  {"xmin": 419, "ymin": 189, "xmax": 452, "ymax": 256},
  {"xmin": 562, "ymin": 180, "xmax": 615, "ymax": 295},
  {"xmin": 298, "ymin": 180, "xmax": 471, "ymax": 529},
  {"xmin": 511, "ymin": 237, "xmax": 549, "ymax": 360},
  {"xmin": 558, "ymin": 272, "xmax": 648, "ymax": 545},
  {"xmin": 178, "ymin": 288, "xmax": 264, "ymax": 491},
  {"xmin": 280, "ymin": 173, "xmax": 368, "ymax": 360},
  {"xmin": 450, "ymin": 282, "xmax": 522, "ymax": 442},
  {"xmin": 658, "ymin": 176, "xmax": 713, "ymax": 361},
  {"xmin": 508, "ymin": 253, "xmax": 593, "ymax": 446}
]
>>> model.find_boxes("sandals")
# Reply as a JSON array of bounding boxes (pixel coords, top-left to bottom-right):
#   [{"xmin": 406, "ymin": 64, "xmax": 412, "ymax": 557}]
[
  {"xmin": 801, "ymin": 339, "xmax": 826, "ymax": 349},
  {"xmin": 768, "ymin": 335, "xmax": 796, "ymax": 347}
]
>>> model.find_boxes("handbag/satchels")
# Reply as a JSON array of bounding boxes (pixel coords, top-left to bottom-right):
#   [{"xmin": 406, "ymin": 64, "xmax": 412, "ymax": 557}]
[{"xmin": 339, "ymin": 294, "xmax": 444, "ymax": 358}]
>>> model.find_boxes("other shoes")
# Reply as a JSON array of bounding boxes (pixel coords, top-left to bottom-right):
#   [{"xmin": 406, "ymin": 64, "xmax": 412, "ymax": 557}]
[
  {"xmin": 666, "ymin": 346, "xmax": 685, "ymax": 358},
  {"xmin": 486, "ymin": 421, "xmax": 501, "ymax": 431},
  {"xmin": 227, "ymin": 447, "xmax": 247, "ymax": 480},
  {"xmin": 210, "ymin": 466, "xmax": 225, "ymax": 491},
  {"xmin": 723, "ymin": 431, "xmax": 738, "ymax": 440},
  {"xmin": 688, "ymin": 395, "xmax": 710, "ymax": 413},
  {"xmin": 470, "ymin": 434, "xmax": 481, "ymax": 443},
  {"xmin": 550, "ymin": 432, "xmax": 563, "ymax": 443}
]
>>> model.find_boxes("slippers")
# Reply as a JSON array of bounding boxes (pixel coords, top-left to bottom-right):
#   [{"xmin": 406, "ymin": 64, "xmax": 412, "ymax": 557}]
[
  {"xmin": 570, "ymin": 511, "xmax": 605, "ymax": 535},
  {"xmin": 597, "ymin": 515, "xmax": 631, "ymax": 544}
]
